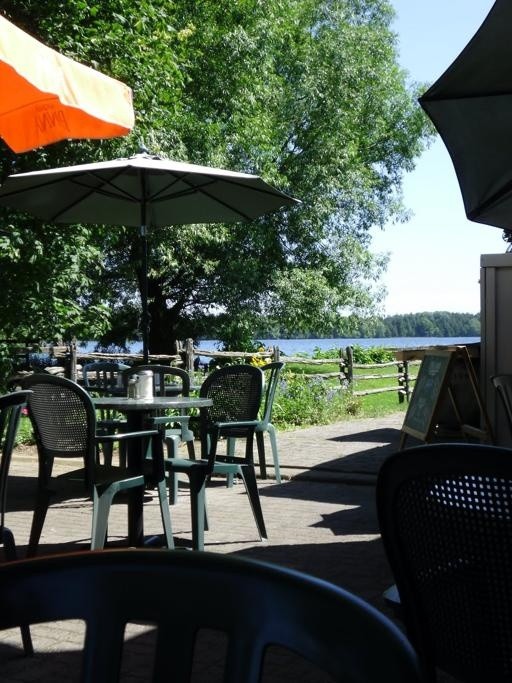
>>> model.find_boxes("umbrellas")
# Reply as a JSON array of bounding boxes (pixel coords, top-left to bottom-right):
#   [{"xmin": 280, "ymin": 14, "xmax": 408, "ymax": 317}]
[
  {"xmin": 0, "ymin": 13, "xmax": 140, "ymax": 153},
  {"xmin": 416, "ymin": 0, "xmax": 512, "ymax": 230},
  {"xmin": 0, "ymin": 150, "xmax": 303, "ymax": 363}
]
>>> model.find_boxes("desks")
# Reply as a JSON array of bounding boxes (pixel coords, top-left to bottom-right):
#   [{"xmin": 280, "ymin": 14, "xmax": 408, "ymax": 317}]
[
  {"xmin": 82, "ymin": 387, "xmax": 213, "ymax": 550},
  {"xmin": 395, "ymin": 349, "xmax": 427, "ymax": 403}
]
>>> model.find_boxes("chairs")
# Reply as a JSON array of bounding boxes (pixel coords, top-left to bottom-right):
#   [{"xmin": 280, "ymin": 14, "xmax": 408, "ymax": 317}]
[
  {"xmin": 0, "ymin": 550, "xmax": 418, "ymax": 683},
  {"xmin": 81, "ymin": 363, "xmax": 133, "ymax": 473},
  {"xmin": 131, "ymin": 361, "xmax": 283, "ymax": 547},
  {"xmin": 375, "ymin": 441, "xmax": 510, "ymax": 682},
  {"xmin": 0, "ymin": 373, "xmax": 147, "ymax": 551}
]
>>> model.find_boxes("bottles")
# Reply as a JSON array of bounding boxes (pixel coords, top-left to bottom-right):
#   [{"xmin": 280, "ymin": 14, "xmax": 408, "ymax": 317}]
[{"xmin": 127, "ymin": 370, "xmax": 153, "ymax": 399}]
